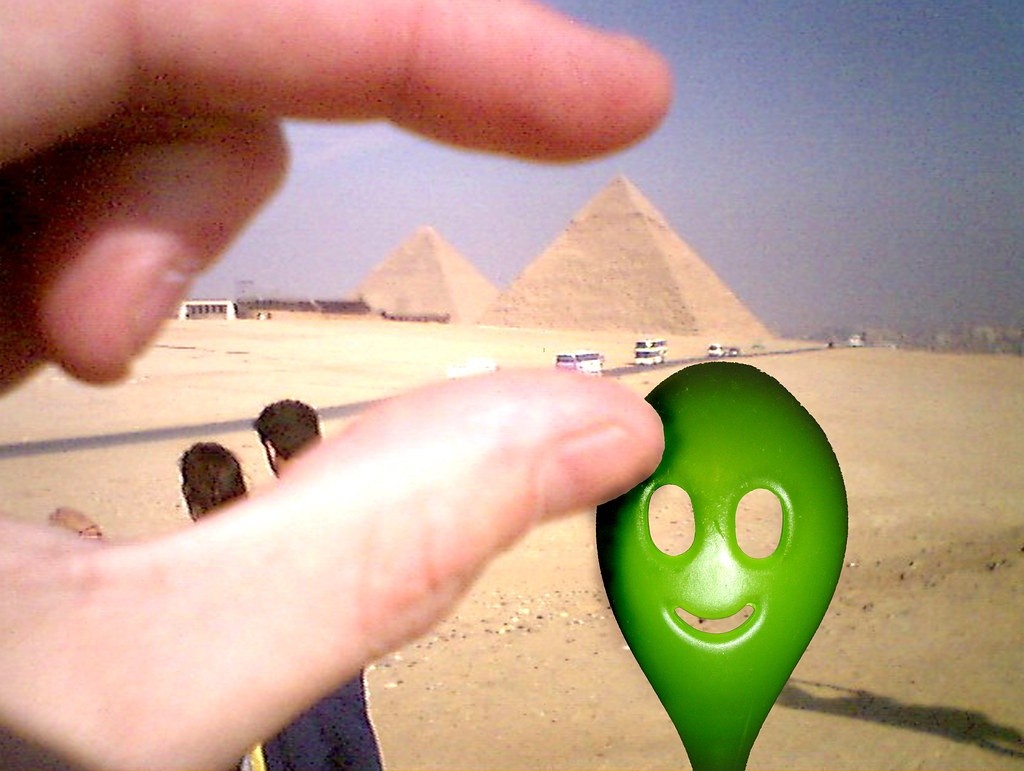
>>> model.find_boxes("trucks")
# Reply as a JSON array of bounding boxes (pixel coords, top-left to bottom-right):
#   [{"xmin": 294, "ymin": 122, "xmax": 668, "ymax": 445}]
[
  {"xmin": 707, "ymin": 342, "xmax": 740, "ymax": 357},
  {"xmin": 849, "ymin": 333, "xmax": 866, "ymax": 348}
]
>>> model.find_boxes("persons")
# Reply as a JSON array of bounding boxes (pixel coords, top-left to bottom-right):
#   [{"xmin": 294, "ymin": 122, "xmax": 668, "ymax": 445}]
[
  {"xmin": 251, "ymin": 398, "xmax": 383, "ymax": 771},
  {"xmin": 177, "ymin": 441, "xmax": 265, "ymax": 771},
  {"xmin": 0, "ymin": 0, "xmax": 674, "ymax": 771}
]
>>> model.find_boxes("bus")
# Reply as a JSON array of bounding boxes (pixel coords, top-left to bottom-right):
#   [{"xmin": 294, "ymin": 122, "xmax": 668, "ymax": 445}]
[
  {"xmin": 630, "ymin": 338, "xmax": 666, "ymax": 366},
  {"xmin": 554, "ymin": 351, "xmax": 604, "ymax": 380}
]
